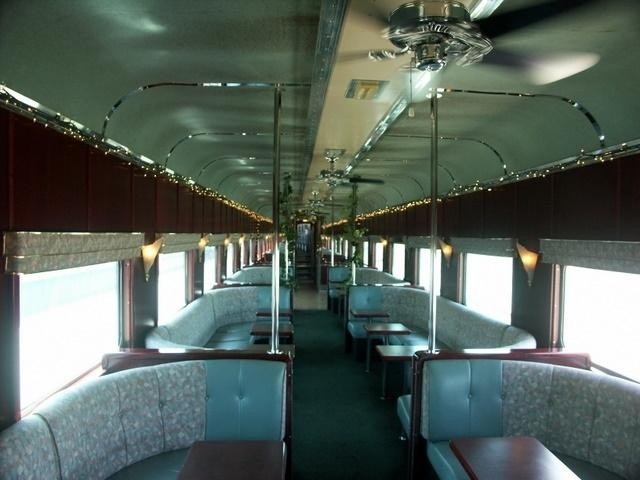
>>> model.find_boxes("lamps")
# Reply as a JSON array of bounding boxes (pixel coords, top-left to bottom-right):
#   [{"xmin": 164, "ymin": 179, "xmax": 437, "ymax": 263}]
[
  {"xmin": 224, "ymin": 234, "xmax": 233, "ymax": 254},
  {"xmin": 379, "ymin": 236, "xmax": 390, "ymax": 253},
  {"xmin": 140, "ymin": 237, "xmax": 165, "ymax": 282},
  {"xmin": 238, "ymin": 233, "xmax": 246, "ymax": 249},
  {"xmin": 438, "ymin": 238, "xmax": 456, "ymax": 270},
  {"xmin": 514, "ymin": 237, "xmax": 542, "ymax": 288},
  {"xmin": 198, "ymin": 234, "xmax": 214, "ymax": 263}
]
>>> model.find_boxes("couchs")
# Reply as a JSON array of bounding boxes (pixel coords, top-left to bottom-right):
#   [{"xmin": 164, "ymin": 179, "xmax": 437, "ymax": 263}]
[
  {"xmin": 410, "ymin": 348, "xmax": 640, "ymax": 479},
  {"xmin": 347, "ymin": 286, "xmax": 537, "ymax": 440},
  {"xmin": 0, "ymin": 347, "xmax": 293, "ymax": 480},
  {"xmin": 329, "ymin": 268, "xmax": 413, "ymax": 299},
  {"xmin": 223, "ymin": 265, "xmax": 272, "ymax": 284},
  {"xmin": 147, "ymin": 285, "xmax": 293, "ymax": 349}
]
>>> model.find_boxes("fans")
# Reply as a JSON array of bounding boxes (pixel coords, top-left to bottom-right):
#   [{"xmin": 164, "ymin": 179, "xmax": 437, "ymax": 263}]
[
  {"xmin": 290, "ymin": 208, "xmax": 330, "ymax": 218},
  {"xmin": 286, "ymin": 190, "xmax": 350, "ymax": 208},
  {"xmin": 291, "ymin": 149, "xmax": 385, "ymax": 186},
  {"xmin": 327, "ymin": 0, "xmax": 639, "ymax": 88}
]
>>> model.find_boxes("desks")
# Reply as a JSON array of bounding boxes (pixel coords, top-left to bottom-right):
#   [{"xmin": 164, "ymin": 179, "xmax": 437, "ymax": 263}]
[
  {"xmin": 350, "ymin": 308, "xmax": 390, "ymax": 374},
  {"xmin": 449, "ymin": 433, "xmax": 583, "ymax": 480},
  {"xmin": 176, "ymin": 438, "xmax": 288, "ymax": 479},
  {"xmin": 256, "ymin": 306, "xmax": 294, "ymax": 317},
  {"xmin": 246, "ymin": 344, "xmax": 296, "ymax": 356},
  {"xmin": 376, "ymin": 345, "xmax": 429, "ymax": 395},
  {"xmin": 361, "ymin": 321, "xmax": 411, "ymax": 401},
  {"xmin": 251, "ymin": 322, "xmax": 294, "ymax": 336}
]
